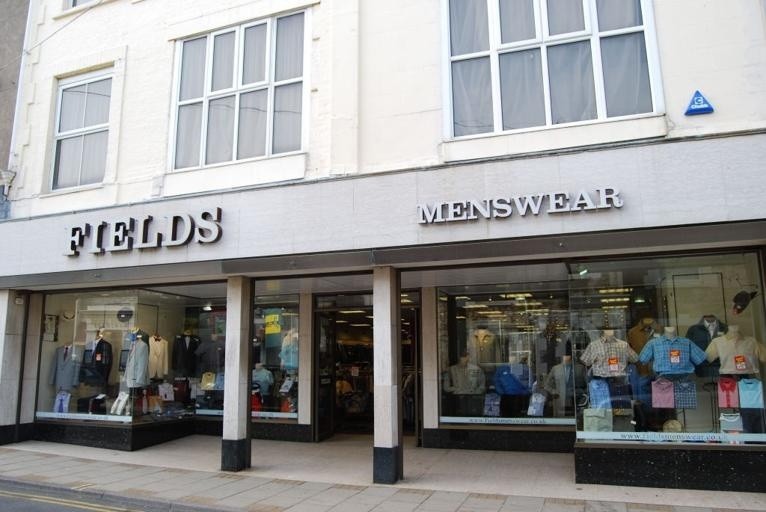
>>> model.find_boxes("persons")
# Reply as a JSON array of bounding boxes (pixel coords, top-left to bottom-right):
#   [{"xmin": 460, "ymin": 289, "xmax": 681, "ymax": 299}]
[
  {"xmin": 48, "ymin": 327, "xmax": 300, "ymax": 396},
  {"xmin": 442, "ymin": 314, "xmax": 766, "ymax": 396}
]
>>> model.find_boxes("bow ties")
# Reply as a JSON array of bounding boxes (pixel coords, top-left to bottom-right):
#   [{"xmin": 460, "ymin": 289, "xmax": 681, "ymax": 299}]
[
  {"xmin": 184, "ymin": 335, "xmax": 191, "ymax": 338},
  {"xmin": 154, "ymin": 338, "xmax": 161, "ymax": 342}
]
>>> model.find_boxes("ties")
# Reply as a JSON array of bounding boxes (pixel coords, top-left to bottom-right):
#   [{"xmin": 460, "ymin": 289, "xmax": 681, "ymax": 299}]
[
  {"xmin": 142, "ymin": 389, "xmax": 148, "ymax": 415},
  {"xmin": 64, "ymin": 347, "xmax": 68, "ymax": 362},
  {"xmin": 129, "ymin": 343, "xmax": 135, "ymax": 356},
  {"xmin": 58, "ymin": 398, "xmax": 64, "ymax": 413},
  {"xmin": 112, "ymin": 399, "xmax": 121, "ymax": 415}
]
